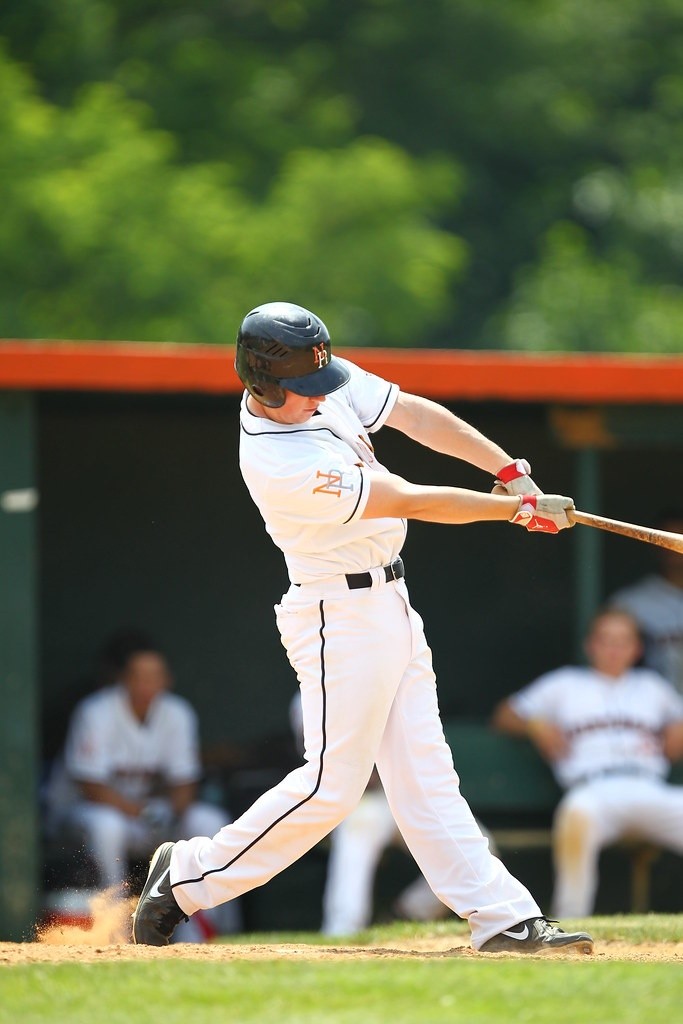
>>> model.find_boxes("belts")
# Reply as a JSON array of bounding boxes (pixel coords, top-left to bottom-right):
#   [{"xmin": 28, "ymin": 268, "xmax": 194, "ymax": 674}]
[{"xmin": 292, "ymin": 556, "xmax": 404, "ymax": 589}]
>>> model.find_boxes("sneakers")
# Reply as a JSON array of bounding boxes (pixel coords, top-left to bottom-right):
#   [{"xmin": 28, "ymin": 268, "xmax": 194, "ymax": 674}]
[
  {"xmin": 478, "ymin": 915, "xmax": 593, "ymax": 954},
  {"xmin": 131, "ymin": 840, "xmax": 190, "ymax": 945}
]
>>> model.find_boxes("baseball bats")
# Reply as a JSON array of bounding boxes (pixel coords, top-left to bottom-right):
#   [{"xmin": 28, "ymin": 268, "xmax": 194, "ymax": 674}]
[{"xmin": 489, "ymin": 479, "xmax": 683, "ymax": 557}]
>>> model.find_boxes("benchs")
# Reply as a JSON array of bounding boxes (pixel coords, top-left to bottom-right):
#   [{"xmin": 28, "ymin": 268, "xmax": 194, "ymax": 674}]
[{"xmin": 446, "ymin": 724, "xmax": 661, "ymax": 913}]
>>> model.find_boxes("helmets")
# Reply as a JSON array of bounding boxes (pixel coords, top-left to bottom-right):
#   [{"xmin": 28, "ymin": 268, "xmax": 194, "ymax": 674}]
[{"xmin": 232, "ymin": 301, "xmax": 351, "ymax": 409}]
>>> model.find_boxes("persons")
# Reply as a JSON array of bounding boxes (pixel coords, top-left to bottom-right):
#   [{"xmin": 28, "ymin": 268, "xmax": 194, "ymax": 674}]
[
  {"xmin": 132, "ymin": 300, "xmax": 595, "ymax": 962},
  {"xmin": 495, "ymin": 607, "xmax": 683, "ymax": 919},
  {"xmin": 290, "ymin": 690, "xmax": 497, "ymax": 937},
  {"xmin": 42, "ymin": 647, "xmax": 233, "ymax": 945}
]
